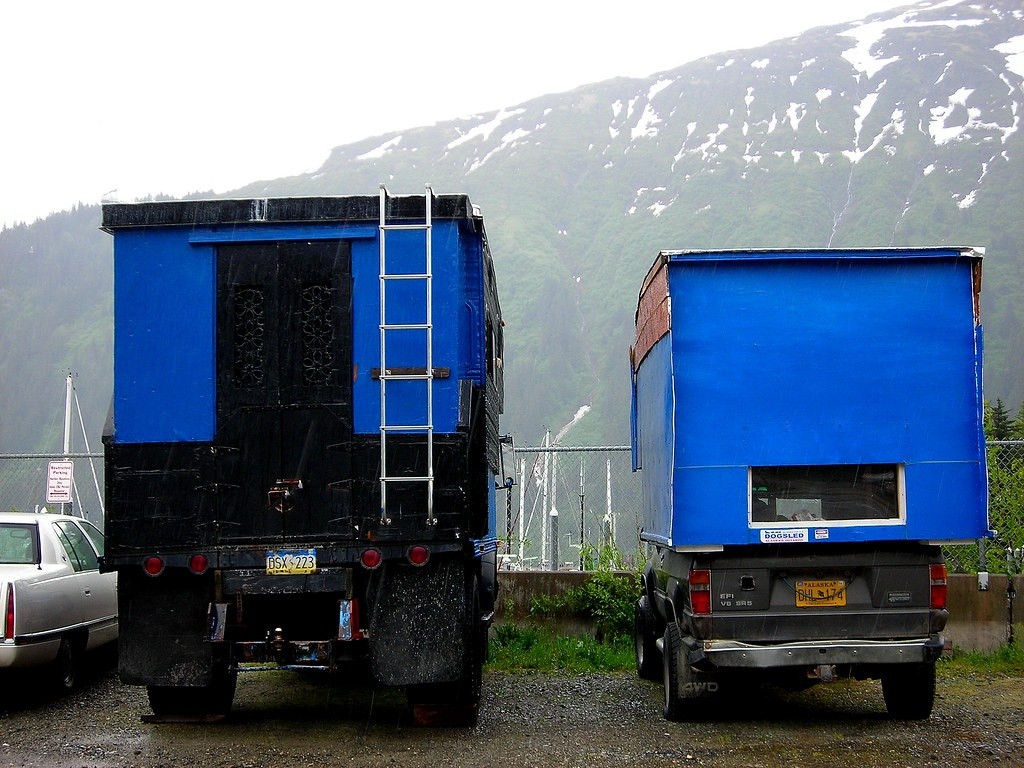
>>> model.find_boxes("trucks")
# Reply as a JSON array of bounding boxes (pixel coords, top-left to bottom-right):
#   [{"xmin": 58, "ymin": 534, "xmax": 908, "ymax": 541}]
[
  {"xmin": 98, "ymin": 205, "xmax": 524, "ymax": 727},
  {"xmin": 629, "ymin": 244, "xmax": 992, "ymax": 728}
]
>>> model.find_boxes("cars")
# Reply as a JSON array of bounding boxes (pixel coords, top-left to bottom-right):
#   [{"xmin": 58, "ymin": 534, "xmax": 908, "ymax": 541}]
[{"xmin": 0, "ymin": 513, "xmax": 120, "ymax": 690}]
